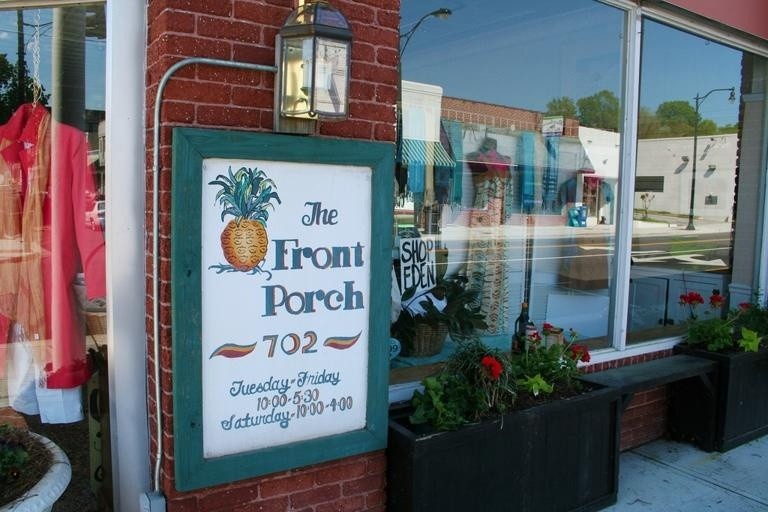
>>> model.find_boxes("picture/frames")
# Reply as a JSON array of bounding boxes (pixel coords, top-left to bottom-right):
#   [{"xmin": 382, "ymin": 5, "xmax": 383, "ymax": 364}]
[{"xmin": 172, "ymin": 126, "xmax": 395, "ymax": 492}]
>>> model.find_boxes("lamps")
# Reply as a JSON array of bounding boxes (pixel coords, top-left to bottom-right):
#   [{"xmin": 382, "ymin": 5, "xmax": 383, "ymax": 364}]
[{"xmin": 272, "ymin": 0, "xmax": 353, "ymax": 134}]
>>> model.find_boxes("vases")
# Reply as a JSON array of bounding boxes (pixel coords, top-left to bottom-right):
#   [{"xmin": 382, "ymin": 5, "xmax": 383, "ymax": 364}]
[
  {"xmin": 666, "ymin": 343, "xmax": 768, "ymax": 452},
  {"xmin": 388, "ymin": 378, "xmax": 623, "ymax": 512}
]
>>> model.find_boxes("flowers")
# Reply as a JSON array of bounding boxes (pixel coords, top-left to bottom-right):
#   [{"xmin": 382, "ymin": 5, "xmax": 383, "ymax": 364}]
[
  {"xmin": 678, "ymin": 286, "xmax": 768, "ymax": 352},
  {"xmin": 408, "ymin": 320, "xmax": 591, "ymax": 433}
]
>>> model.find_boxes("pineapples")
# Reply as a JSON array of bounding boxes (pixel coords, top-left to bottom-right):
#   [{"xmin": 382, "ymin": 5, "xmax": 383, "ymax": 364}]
[{"xmin": 221, "ymin": 219, "xmax": 268, "ymax": 272}]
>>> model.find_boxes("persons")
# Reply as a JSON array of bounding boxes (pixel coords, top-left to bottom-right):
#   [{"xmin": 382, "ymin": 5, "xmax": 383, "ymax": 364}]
[{"xmin": 465, "ymin": 137, "xmax": 513, "ymax": 333}]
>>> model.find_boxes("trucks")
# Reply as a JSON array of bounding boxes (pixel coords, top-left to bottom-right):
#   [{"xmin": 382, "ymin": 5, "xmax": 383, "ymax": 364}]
[{"xmin": 80, "ymin": 197, "xmax": 109, "ymax": 236}]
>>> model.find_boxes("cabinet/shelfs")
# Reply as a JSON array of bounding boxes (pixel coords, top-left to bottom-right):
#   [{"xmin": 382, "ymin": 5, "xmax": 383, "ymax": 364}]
[{"xmin": 627, "ymin": 274, "xmax": 723, "ymax": 336}]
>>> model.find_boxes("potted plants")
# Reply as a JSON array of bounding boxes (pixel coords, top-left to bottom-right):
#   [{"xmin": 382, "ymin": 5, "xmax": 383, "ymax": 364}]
[
  {"xmin": 394, "ymin": 225, "xmax": 489, "ymax": 356},
  {"xmin": 0, "ymin": 422, "xmax": 72, "ymax": 512}
]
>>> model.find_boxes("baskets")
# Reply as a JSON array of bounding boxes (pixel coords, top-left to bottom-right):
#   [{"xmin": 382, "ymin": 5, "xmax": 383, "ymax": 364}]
[{"xmin": 391, "ymin": 321, "xmax": 448, "ymax": 356}]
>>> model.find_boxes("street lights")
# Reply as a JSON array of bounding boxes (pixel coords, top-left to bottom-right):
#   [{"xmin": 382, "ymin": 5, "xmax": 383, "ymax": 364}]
[
  {"xmin": 397, "ymin": 8, "xmax": 453, "ymax": 166},
  {"xmin": 687, "ymin": 85, "xmax": 736, "ymax": 231}
]
